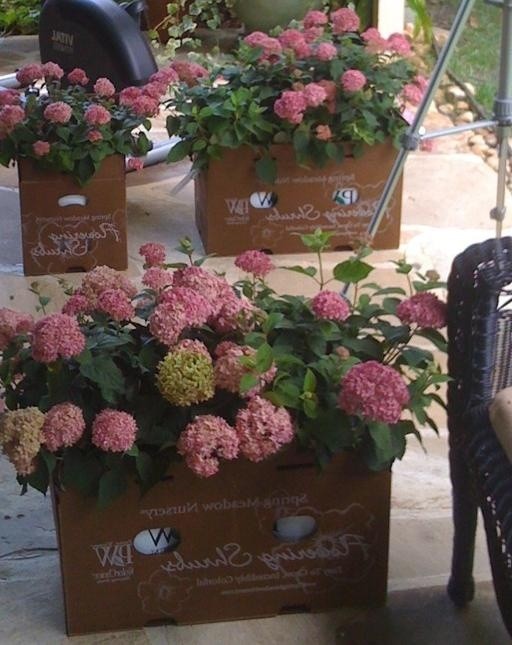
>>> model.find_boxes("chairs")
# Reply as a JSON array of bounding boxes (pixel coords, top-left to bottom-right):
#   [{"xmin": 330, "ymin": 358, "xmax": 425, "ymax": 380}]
[
  {"xmin": 1, "ymin": 1, "xmax": 234, "ymax": 178},
  {"xmin": 444, "ymin": 235, "xmax": 512, "ymax": 640}
]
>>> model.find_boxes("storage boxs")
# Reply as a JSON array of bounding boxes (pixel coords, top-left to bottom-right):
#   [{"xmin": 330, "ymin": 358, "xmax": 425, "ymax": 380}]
[
  {"xmin": 17, "ymin": 155, "xmax": 129, "ymax": 277},
  {"xmin": 193, "ymin": 136, "xmax": 404, "ymax": 257}
]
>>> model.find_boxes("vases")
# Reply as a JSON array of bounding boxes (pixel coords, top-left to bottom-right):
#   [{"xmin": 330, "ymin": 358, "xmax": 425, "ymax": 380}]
[{"xmin": 39, "ymin": 461, "xmax": 396, "ymax": 640}]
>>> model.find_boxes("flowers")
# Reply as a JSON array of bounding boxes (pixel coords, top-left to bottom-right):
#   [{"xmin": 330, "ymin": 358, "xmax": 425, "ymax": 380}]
[
  {"xmin": 2, "ymin": 242, "xmax": 450, "ymax": 483},
  {"xmin": 0, "ymin": 57, "xmax": 211, "ymax": 172},
  {"xmin": 244, "ymin": 5, "xmax": 438, "ymax": 162}
]
